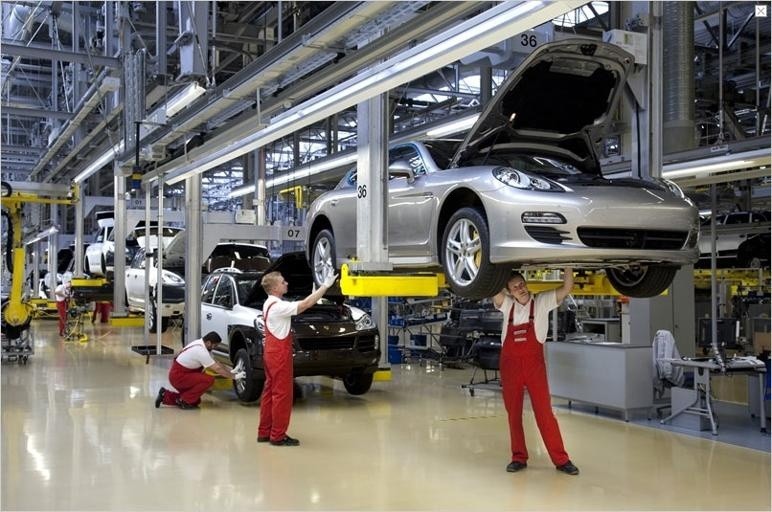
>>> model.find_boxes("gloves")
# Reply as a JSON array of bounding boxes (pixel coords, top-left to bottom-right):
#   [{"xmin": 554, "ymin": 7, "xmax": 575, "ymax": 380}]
[
  {"xmin": 235, "ymin": 370, "xmax": 247, "ymax": 380},
  {"xmin": 230, "ymin": 365, "xmax": 240, "ymax": 373},
  {"xmin": 324, "ymin": 267, "xmax": 339, "ymax": 288}
]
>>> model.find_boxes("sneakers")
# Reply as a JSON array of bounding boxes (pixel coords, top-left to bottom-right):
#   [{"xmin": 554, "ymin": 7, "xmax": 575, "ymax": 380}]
[
  {"xmin": 506, "ymin": 460, "xmax": 527, "ymax": 472},
  {"xmin": 258, "ymin": 437, "xmax": 271, "ymax": 442},
  {"xmin": 556, "ymin": 461, "xmax": 578, "ymax": 475},
  {"xmin": 271, "ymin": 436, "xmax": 300, "ymax": 446}
]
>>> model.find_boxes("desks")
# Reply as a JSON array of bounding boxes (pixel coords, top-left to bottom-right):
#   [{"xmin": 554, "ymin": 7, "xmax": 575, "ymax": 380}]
[{"xmin": 660, "ymin": 356, "xmax": 768, "ymax": 435}]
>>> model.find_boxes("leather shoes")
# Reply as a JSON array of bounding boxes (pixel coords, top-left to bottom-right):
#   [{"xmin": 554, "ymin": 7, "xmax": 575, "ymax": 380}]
[
  {"xmin": 176, "ymin": 398, "xmax": 192, "ymax": 409},
  {"xmin": 155, "ymin": 387, "xmax": 165, "ymax": 408}
]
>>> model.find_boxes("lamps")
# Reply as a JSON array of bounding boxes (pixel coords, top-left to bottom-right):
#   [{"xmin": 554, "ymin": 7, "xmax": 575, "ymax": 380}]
[
  {"xmin": 213, "ymin": 183, "xmax": 257, "ymax": 204},
  {"xmin": 265, "ymin": 148, "xmax": 357, "ymax": 189},
  {"xmin": 164, "ymin": 2, "xmax": 592, "ymax": 187},
  {"xmin": 601, "ymin": 147, "xmax": 771, "ymax": 180},
  {"xmin": 24, "ymin": 225, "xmax": 59, "ymax": 247},
  {"xmin": 73, "ymin": 82, "xmax": 206, "ymax": 185},
  {"xmin": 389, "ymin": 112, "xmax": 480, "ymax": 152},
  {"xmin": 698, "ymin": 208, "xmax": 711, "ymax": 218}
]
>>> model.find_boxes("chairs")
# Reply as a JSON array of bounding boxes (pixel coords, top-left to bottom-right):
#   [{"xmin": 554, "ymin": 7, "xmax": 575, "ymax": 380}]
[{"xmin": 653, "ymin": 329, "xmax": 710, "ymax": 416}]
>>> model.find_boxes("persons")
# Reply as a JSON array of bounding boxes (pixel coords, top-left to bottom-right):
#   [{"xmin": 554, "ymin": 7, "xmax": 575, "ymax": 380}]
[
  {"xmin": 155, "ymin": 331, "xmax": 246, "ymax": 409},
  {"xmin": 257, "ymin": 266, "xmax": 338, "ymax": 446},
  {"xmin": 492, "ymin": 267, "xmax": 580, "ymax": 475},
  {"xmin": 54, "ymin": 281, "xmax": 71, "ymax": 336},
  {"xmin": 101, "ymin": 301, "xmax": 111, "ymax": 323},
  {"xmin": 92, "ymin": 301, "xmax": 101, "ymax": 322}
]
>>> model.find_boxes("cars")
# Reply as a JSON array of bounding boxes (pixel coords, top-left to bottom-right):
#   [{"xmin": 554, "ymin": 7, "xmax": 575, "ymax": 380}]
[
  {"xmin": 302, "ymin": 38, "xmax": 705, "ymax": 299},
  {"xmin": 696, "ymin": 209, "xmax": 771, "ymax": 268}
]
